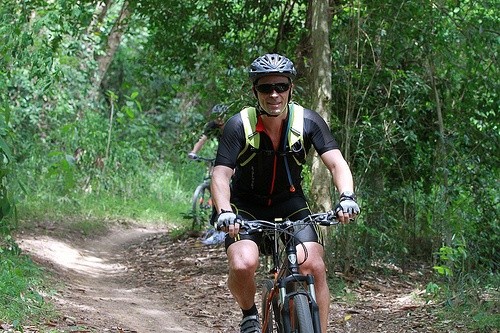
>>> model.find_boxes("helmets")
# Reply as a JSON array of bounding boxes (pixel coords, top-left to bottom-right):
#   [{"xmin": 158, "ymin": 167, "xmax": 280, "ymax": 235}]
[
  {"xmin": 248, "ymin": 53, "xmax": 296, "ymax": 82},
  {"xmin": 213, "ymin": 104, "xmax": 228, "ymax": 113}
]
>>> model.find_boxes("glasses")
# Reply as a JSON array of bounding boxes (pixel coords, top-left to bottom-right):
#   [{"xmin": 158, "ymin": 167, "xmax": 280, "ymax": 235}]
[{"xmin": 254, "ymin": 82, "xmax": 291, "ymax": 93}]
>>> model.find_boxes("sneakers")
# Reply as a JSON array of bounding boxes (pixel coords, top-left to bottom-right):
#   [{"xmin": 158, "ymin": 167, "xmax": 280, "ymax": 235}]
[{"xmin": 240, "ymin": 314, "xmax": 260, "ymax": 333}]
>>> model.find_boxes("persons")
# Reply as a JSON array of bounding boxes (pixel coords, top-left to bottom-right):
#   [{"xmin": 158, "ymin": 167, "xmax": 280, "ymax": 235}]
[
  {"xmin": 189, "ymin": 104, "xmax": 232, "ymax": 226},
  {"xmin": 210, "ymin": 54, "xmax": 360, "ymax": 333}
]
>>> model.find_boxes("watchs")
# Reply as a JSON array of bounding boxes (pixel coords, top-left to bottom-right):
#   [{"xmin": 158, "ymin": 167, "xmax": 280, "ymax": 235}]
[{"xmin": 339, "ymin": 191, "xmax": 357, "ymax": 200}]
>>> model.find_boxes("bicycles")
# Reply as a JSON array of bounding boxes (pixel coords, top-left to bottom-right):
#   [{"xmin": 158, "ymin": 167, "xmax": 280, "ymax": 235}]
[
  {"xmin": 188, "ymin": 154, "xmax": 217, "ymax": 227},
  {"xmin": 217, "ymin": 208, "xmax": 357, "ymax": 333}
]
